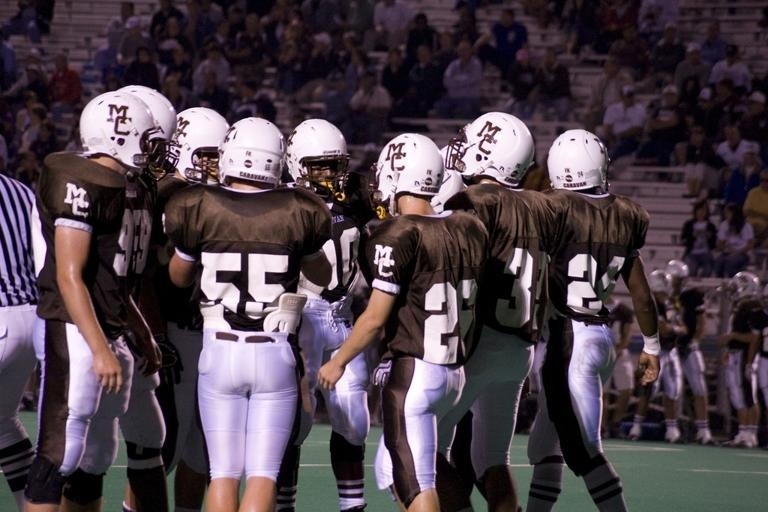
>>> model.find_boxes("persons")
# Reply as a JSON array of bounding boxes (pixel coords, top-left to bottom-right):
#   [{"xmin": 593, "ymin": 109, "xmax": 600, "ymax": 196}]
[
  {"xmin": 732, "ymin": 92, "xmax": 768, "ymax": 153},
  {"xmin": 276, "ymin": 117, "xmax": 374, "ymax": 512},
  {"xmin": 232, "ymin": 77, "xmax": 276, "ymax": 123},
  {"xmin": 6, "ymin": 0, "xmax": 56, "ymax": 45},
  {"xmin": 205, "ymin": 19, "xmax": 231, "ymax": 51},
  {"xmin": 316, "ymin": 133, "xmax": 491, "ymax": 511},
  {"xmin": 635, "ymin": 87, "xmax": 686, "ymax": 159},
  {"xmin": 627, "ymin": 269, "xmax": 686, "ymax": 442},
  {"xmin": 406, "ymin": 46, "xmax": 444, "ymax": 117},
  {"xmin": 2, "ymin": 47, "xmax": 48, "ymax": 147},
  {"xmin": 603, "ymin": 85, "xmax": 647, "ymax": 159},
  {"xmin": 305, "ymin": 34, "xmax": 335, "ymax": 80},
  {"xmin": 62, "ymin": 84, "xmax": 184, "ymax": 512},
  {"xmin": 170, "ymin": 117, "xmax": 333, "ymax": 511},
  {"xmin": 47, "ymin": 54, "xmax": 81, "ymax": 109},
  {"xmin": 673, "ymin": 43, "xmax": 712, "ymax": 88},
  {"xmin": 599, "ymin": 294, "xmax": 637, "ymax": 442},
  {"xmin": 340, "ymin": 31, "xmax": 370, "ymax": 79},
  {"xmin": 653, "ymin": 261, "xmax": 714, "ymax": 445},
  {"xmin": 302, "ymin": 2, "xmax": 328, "ymax": 35},
  {"xmin": 25, "ymin": 92, "xmax": 170, "ymax": 512},
  {"xmin": 596, "ymin": 55, "xmax": 632, "ymax": 117},
  {"xmin": 333, "ymin": 0, "xmax": 355, "ymax": 32},
  {"xmin": 15, "ymin": 147, "xmax": 42, "ymax": 191},
  {"xmin": 724, "ymin": 153, "xmax": 760, "ymax": 229},
  {"xmin": 436, "ymin": 112, "xmax": 557, "ymax": 512},
  {"xmin": 718, "ymin": 123, "xmax": 761, "ymax": 174},
  {"xmin": 682, "ymin": 127, "xmax": 729, "ymax": 197},
  {"xmin": 229, "ymin": 32, "xmax": 265, "ymax": 75},
  {"xmin": 528, "ymin": 46, "xmax": 573, "ymax": 119},
  {"xmin": 653, "ymin": 28, "xmax": 680, "ymax": 60},
  {"xmin": 372, "ymin": 0, "xmax": 408, "ymax": 44},
  {"xmin": 272, "ymin": 41, "xmax": 308, "ymax": 96},
  {"xmin": 273, "ymin": 11, "xmax": 305, "ymax": 50},
  {"xmin": 709, "ymin": 79, "xmax": 747, "ymax": 118},
  {"xmin": 350, "ymin": 70, "xmax": 391, "ymax": 125},
  {"xmin": 121, "ymin": 107, "xmax": 230, "ymax": 511},
  {"xmin": 686, "ymin": 87, "xmax": 724, "ymax": 147},
  {"xmin": 453, "ymin": 5, "xmax": 481, "ymax": 50},
  {"xmin": 700, "ymin": 19, "xmax": 733, "ymax": 55},
  {"xmin": 610, "ymin": 20, "xmax": 654, "ymax": 79},
  {"xmin": 322, "ymin": 72, "xmax": 355, "ymax": 122},
  {"xmin": 381, "ymin": 46, "xmax": 406, "ymax": 98},
  {"xmin": 0, "ymin": 134, "xmax": 47, "ymax": 512},
  {"xmin": 224, "ymin": 15, "xmax": 261, "ymax": 62},
  {"xmin": 195, "ymin": 45, "xmax": 230, "ymax": 91},
  {"xmin": 715, "ymin": 203, "xmax": 754, "ymax": 275},
  {"xmin": 443, "ymin": 42, "xmax": 483, "ymax": 118},
  {"xmin": 190, "ymin": 2, "xmax": 222, "ymax": 32},
  {"xmin": 192, "ymin": 69, "xmax": 234, "ymax": 117},
  {"xmin": 742, "ymin": 167, "xmax": 768, "ymax": 242},
  {"xmin": 510, "ymin": 46, "xmax": 539, "ymax": 108},
  {"xmin": 680, "ymin": 202, "xmax": 718, "ymax": 272},
  {"xmin": 94, "ymin": 0, "xmax": 191, "ymax": 89},
  {"xmin": 719, "ymin": 272, "xmax": 761, "ymax": 450},
  {"xmin": 472, "ymin": 7, "xmax": 528, "ymax": 69},
  {"xmin": 403, "ymin": 13, "xmax": 441, "ymax": 50},
  {"xmin": 526, "ymin": 129, "xmax": 660, "ymax": 511},
  {"xmin": 709, "ymin": 45, "xmax": 753, "ymax": 87}
]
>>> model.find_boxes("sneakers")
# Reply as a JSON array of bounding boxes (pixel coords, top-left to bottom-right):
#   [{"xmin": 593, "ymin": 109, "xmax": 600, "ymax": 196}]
[
  {"xmin": 695, "ymin": 429, "xmax": 716, "ymax": 445},
  {"xmin": 625, "ymin": 424, "xmax": 642, "ymax": 441},
  {"xmin": 721, "ymin": 433, "xmax": 760, "ymax": 449},
  {"xmin": 665, "ymin": 426, "xmax": 684, "ymax": 445}
]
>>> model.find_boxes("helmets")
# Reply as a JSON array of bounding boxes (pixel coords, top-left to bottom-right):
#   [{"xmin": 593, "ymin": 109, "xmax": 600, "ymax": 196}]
[
  {"xmin": 78, "ymin": 90, "xmax": 164, "ymax": 182},
  {"xmin": 429, "ymin": 141, "xmax": 468, "ymax": 216},
  {"xmin": 164, "ymin": 106, "xmax": 231, "ymax": 186},
  {"xmin": 285, "ymin": 117, "xmax": 352, "ymax": 203},
  {"xmin": 374, "ymin": 132, "xmax": 444, "ymax": 216},
  {"xmin": 216, "ymin": 116, "xmax": 288, "ymax": 189},
  {"xmin": 729, "ymin": 271, "xmax": 760, "ymax": 310},
  {"xmin": 115, "ymin": 84, "xmax": 183, "ymax": 185},
  {"xmin": 546, "ymin": 127, "xmax": 612, "ymax": 194},
  {"xmin": 667, "ymin": 260, "xmax": 688, "ymax": 280},
  {"xmin": 444, "ymin": 111, "xmax": 537, "ymax": 188},
  {"xmin": 647, "ymin": 269, "xmax": 674, "ymax": 299}
]
